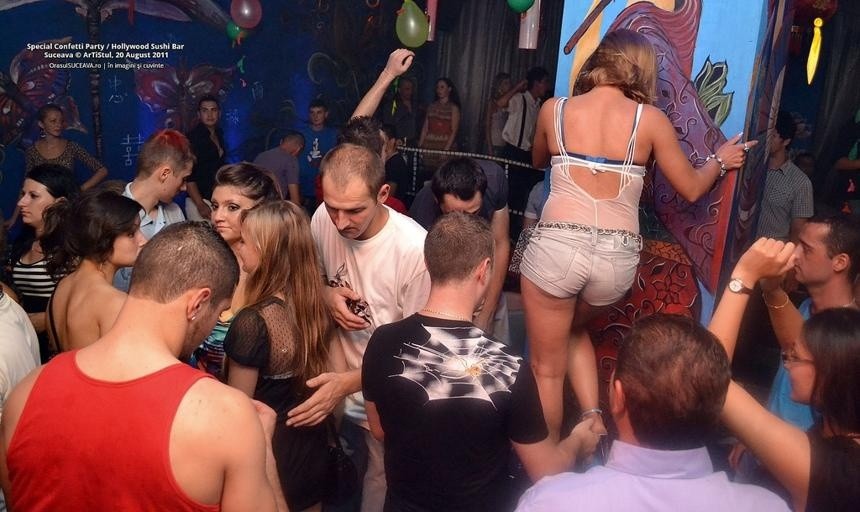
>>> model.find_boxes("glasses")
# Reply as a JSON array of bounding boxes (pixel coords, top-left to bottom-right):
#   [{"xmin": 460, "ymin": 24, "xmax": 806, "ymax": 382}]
[{"xmin": 782, "ymin": 348, "xmax": 815, "ymax": 364}]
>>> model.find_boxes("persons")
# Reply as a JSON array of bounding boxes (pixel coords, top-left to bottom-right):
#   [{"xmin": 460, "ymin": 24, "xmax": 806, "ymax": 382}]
[
  {"xmin": 362, "ymin": 210, "xmax": 601, "ymax": 511},
  {"xmin": 0, "ymin": 275, "xmax": 42, "ymax": 512},
  {"xmin": 1, "ymin": 25, "xmax": 859, "ymax": 512},
  {"xmin": 706, "ymin": 235, "xmax": 859, "ymax": 512},
  {"xmin": 0, "ymin": 219, "xmax": 289, "ymax": 512},
  {"xmin": 285, "ymin": 141, "xmax": 432, "ymax": 511},
  {"xmin": 511, "ymin": 313, "xmax": 794, "ymax": 512},
  {"xmin": 222, "ymin": 201, "xmax": 330, "ymax": 512}
]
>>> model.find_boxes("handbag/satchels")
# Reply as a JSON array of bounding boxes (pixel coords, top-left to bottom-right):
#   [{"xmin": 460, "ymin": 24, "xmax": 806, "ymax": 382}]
[{"xmin": 329, "ymin": 445, "xmax": 355, "ymax": 503}]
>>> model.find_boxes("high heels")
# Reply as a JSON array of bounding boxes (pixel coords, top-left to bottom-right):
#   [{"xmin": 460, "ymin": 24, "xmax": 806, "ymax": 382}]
[{"xmin": 580, "ymin": 409, "xmax": 609, "ymax": 465}]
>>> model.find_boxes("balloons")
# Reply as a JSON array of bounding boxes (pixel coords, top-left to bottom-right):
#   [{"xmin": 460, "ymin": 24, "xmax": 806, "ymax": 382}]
[{"xmin": 229, "ymin": 0, "xmax": 538, "ymax": 48}]
